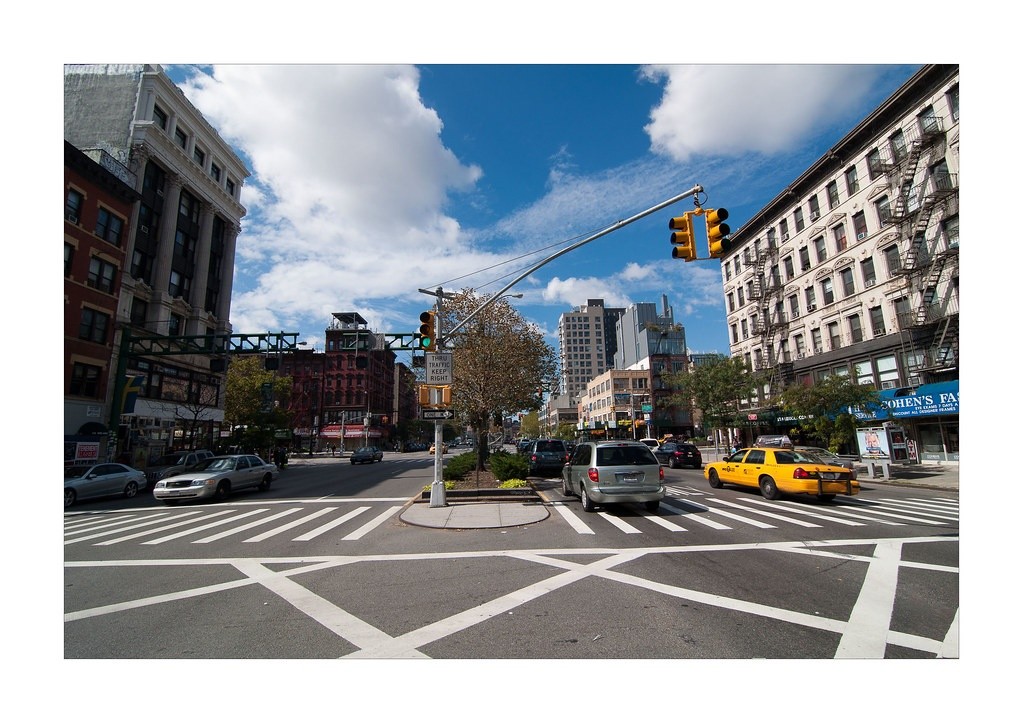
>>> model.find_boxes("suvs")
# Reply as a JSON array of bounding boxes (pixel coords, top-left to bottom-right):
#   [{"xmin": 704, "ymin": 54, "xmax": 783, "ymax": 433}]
[{"xmin": 152, "ymin": 449, "xmax": 219, "ymax": 485}]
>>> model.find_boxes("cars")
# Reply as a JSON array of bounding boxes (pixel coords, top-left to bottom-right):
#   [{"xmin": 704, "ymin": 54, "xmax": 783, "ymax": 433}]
[
  {"xmin": 704, "ymin": 435, "xmax": 860, "ymax": 501},
  {"xmin": 430, "ymin": 442, "xmax": 449, "ymax": 454},
  {"xmin": 562, "ymin": 441, "xmax": 667, "ymax": 511},
  {"xmin": 350, "ymin": 445, "xmax": 383, "ymax": 464},
  {"xmin": 152, "ymin": 454, "xmax": 276, "ymax": 506},
  {"xmin": 653, "ymin": 441, "xmax": 702, "ymax": 469},
  {"xmin": 522, "ymin": 439, "xmax": 569, "ymax": 474},
  {"xmin": 639, "ymin": 439, "xmax": 660, "ymax": 454},
  {"xmin": 64, "ymin": 463, "xmax": 149, "ymax": 509},
  {"xmin": 516, "ymin": 438, "xmax": 530, "ymax": 451}
]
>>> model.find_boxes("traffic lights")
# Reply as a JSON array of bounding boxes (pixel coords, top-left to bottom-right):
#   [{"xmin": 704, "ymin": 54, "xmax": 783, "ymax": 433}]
[
  {"xmin": 419, "ymin": 311, "xmax": 435, "ymax": 351},
  {"xmin": 610, "ymin": 406, "xmax": 616, "ymax": 413},
  {"xmin": 705, "ymin": 208, "xmax": 732, "ymax": 257},
  {"xmin": 669, "ymin": 212, "xmax": 697, "ymax": 262}
]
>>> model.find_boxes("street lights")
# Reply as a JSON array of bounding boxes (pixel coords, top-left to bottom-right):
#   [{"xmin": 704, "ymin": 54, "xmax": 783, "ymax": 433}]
[{"xmin": 484, "ymin": 292, "xmax": 524, "ymax": 324}]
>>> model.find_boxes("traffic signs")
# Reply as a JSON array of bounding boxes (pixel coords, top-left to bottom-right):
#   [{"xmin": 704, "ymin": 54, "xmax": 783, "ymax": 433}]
[{"xmin": 423, "ymin": 408, "xmax": 455, "ymax": 420}]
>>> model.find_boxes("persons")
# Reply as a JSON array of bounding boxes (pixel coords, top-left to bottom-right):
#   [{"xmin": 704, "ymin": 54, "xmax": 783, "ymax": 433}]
[
  {"xmin": 114, "ymin": 442, "xmax": 400, "ymax": 471},
  {"xmin": 732, "ymin": 436, "xmax": 742, "ymax": 452},
  {"xmin": 865, "ymin": 432, "xmax": 889, "ymax": 456}
]
